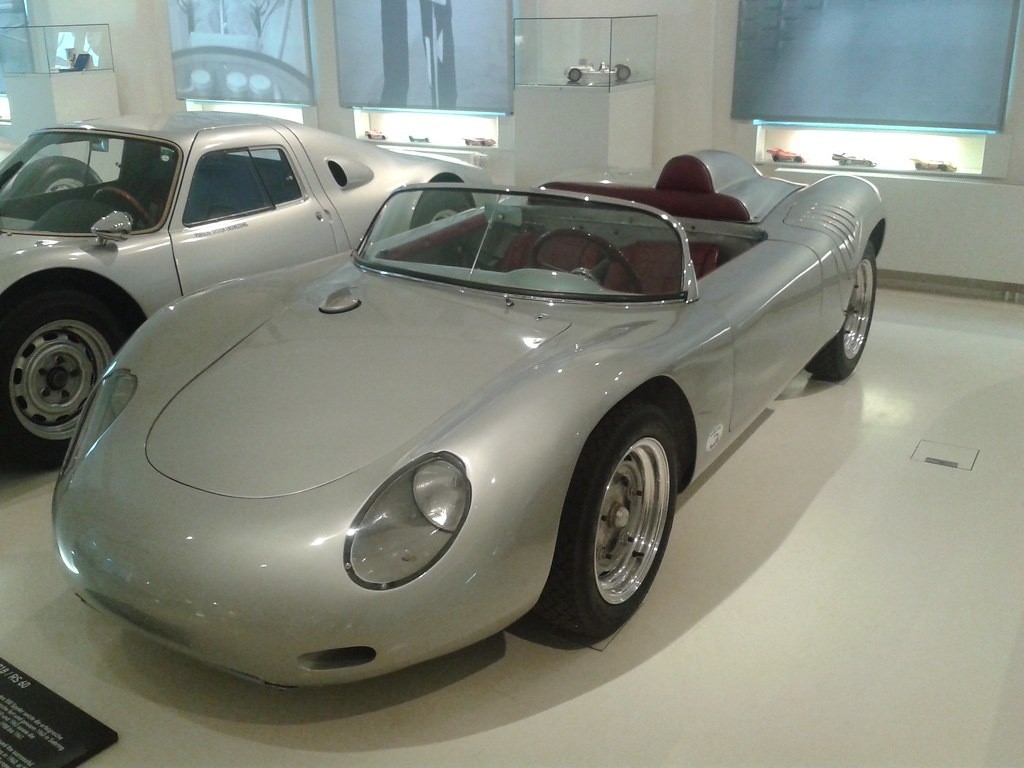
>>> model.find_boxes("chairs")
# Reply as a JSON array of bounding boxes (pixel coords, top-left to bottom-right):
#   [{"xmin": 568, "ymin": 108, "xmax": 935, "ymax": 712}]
[
  {"xmin": 142, "ymin": 150, "xmax": 240, "ymax": 233},
  {"xmin": 89, "ymin": 151, "xmax": 169, "ymax": 229},
  {"xmin": 240, "ymin": 163, "xmax": 301, "ymax": 214},
  {"xmin": 492, "ymin": 180, "xmax": 622, "ymax": 285},
  {"xmin": 603, "ymin": 154, "xmax": 752, "ymax": 301}
]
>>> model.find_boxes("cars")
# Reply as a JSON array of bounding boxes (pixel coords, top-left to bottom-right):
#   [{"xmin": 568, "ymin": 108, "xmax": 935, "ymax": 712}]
[
  {"xmin": 464, "ymin": 137, "xmax": 495, "ymax": 148},
  {"xmin": 2, "ymin": 117, "xmax": 494, "ymax": 473},
  {"xmin": 831, "ymin": 154, "xmax": 877, "ymax": 168},
  {"xmin": 565, "ymin": 58, "xmax": 631, "ymax": 81},
  {"xmin": 769, "ymin": 146, "xmax": 806, "ymax": 164},
  {"xmin": 410, "ymin": 135, "xmax": 430, "ymax": 142},
  {"xmin": 366, "ymin": 129, "xmax": 387, "ymax": 141},
  {"xmin": 54, "ymin": 150, "xmax": 885, "ymax": 681},
  {"xmin": 912, "ymin": 158, "xmax": 958, "ymax": 173}
]
